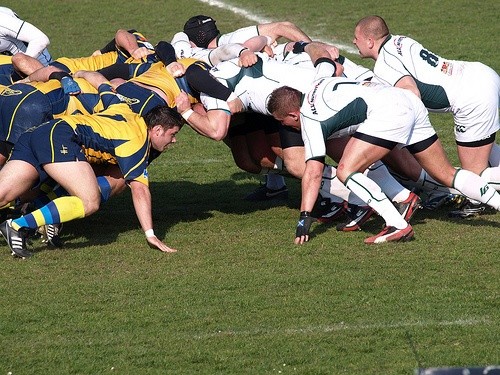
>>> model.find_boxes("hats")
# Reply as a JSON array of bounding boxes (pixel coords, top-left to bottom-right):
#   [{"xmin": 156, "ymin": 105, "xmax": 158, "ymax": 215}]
[{"xmin": 184, "ymin": 15, "xmax": 220, "ymax": 49}]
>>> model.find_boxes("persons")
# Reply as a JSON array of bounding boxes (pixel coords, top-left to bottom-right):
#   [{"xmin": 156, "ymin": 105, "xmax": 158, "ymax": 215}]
[
  {"xmin": 0, "ymin": 6, "xmax": 497, "ymax": 243},
  {"xmin": 0, "ymin": 70, "xmax": 186, "ymax": 257},
  {"xmin": 267, "ymin": 77, "xmax": 500, "ymax": 245},
  {"xmin": 353, "ymin": 16, "xmax": 500, "ymax": 192}
]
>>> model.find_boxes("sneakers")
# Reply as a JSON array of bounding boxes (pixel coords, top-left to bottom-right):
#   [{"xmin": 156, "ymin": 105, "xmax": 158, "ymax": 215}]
[
  {"xmin": 336, "ymin": 206, "xmax": 379, "ymax": 232},
  {"xmin": 448, "ymin": 200, "xmax": 487, "ymax": 217},
  {"xmin": 423, "ymin": 193, "xmax": 455, "ymax": 210},
  {"xmin": 318, "ymin": 200, "xmax": 348, "ymax": 222},
  {"xmin": 363, "ymin": 223, "xmax": 414, "ymax": 244},
  {"xmin": 7, "ymin": 203, "xmax": 33, "ymax": 246},
  {"xmin": 0, "ymin": 219, "xmax": 30, "ymax": 258},
  {"xmin": 381, "ymin": 191, "xmax": 423, "ymax": 229},
  {"xmin": 33, "ymin": 195, "xmax": 63, "ymax": 248},
  {"xmin": 247, "ymin": 183, "xmax": 289, "ymax": 200}
]
